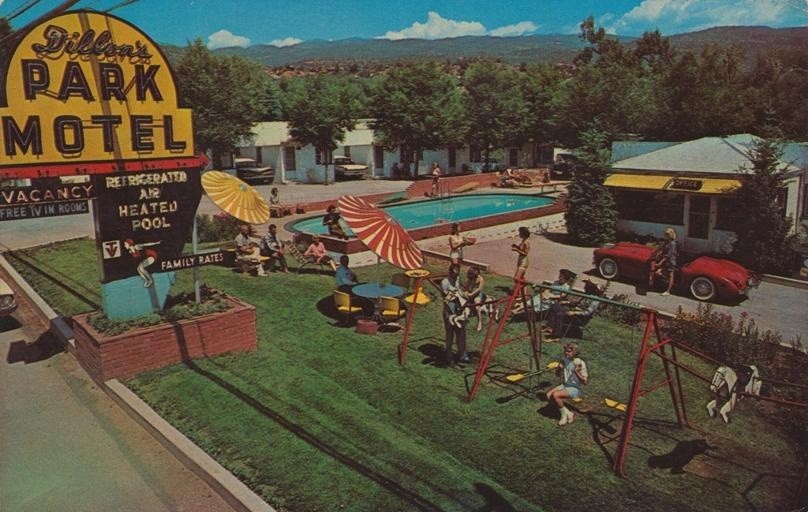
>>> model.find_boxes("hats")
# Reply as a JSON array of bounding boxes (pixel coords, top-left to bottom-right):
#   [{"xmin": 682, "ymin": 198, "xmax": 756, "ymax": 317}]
[{"xmin": 582, "ymin": 277, "xmax": 601, "ymax": 290}]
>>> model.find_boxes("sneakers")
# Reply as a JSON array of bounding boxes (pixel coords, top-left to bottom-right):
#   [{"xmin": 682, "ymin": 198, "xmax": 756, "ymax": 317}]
[{"xmin": 660, "ymin": 291, "xmax": 670, "ymax": 295}]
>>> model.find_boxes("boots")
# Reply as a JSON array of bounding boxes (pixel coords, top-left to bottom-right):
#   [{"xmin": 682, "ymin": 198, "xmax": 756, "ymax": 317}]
[{"xmin": 558, "ymin": 406, "xmax": 574, "ymax": 426}]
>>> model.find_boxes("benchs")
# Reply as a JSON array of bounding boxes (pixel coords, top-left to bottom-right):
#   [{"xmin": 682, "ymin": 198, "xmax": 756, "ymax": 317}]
[
  {"xmin": 270, "ymin": 202, "xmax": 309, "ymax": 215},
  {"xmin": 234, "ymin": 255, "xmax": 271, "ymax": 262}
]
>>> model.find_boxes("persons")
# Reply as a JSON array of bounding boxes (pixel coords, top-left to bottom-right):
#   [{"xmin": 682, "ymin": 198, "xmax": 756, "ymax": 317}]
[
  {"xmin": 401, "ymin": 160, "xmax": 411, "ymax": 178},
  {"xmin": 511, "ymin": 227, "xmax": 529, "ymax": 286},
  {"xmin": 270, "ymin": 188, "xmax": 286, "ymax": 209},
  {"xmin": 546, "ymin": 343, "xmax": 588, "ymax": 426},
  {"xmin": 233, "ymin": 226, "xmax": 266, "ymax": 276},
  {"xmin": 260, "ymin": 224, "xmax": 291, "ymax": 274},
  {"xmin": 304, "ymin": 233, "xmax": 336, "ymax": 274},
  {"xmin": 514, "ymin": 270, "xmax": 570, "ymax": 312},
  {"xmin": 465, "ymin": 266, "xmax": 484, "ymax": 331},
  {"xmin": 656, "ymin": 228, "xmax": 677, "ymax": 296},
  {"xmin": 442, "ymin": 264, "xmax": 470, "ymax": 363},
  {"xmin": 324, "ymin": 205, "xmax": 347, "ymax": 240},
  {"xmin": 431, "ymin": 162, "xmax": 440, "ymax": 196},
  {"xmin": 494, "ymin": 166, "xmax": 535, "ymax": 188},
  {"xmin": 542, "ymin": 284, "xmax": 598, "ymax": 334},
  {"xmin": 336, "ymin": 256, "xmax": 360, "ymax": 300},
  {"xmin": 449, "ymin": 222, "xmax": 464, "ymax": 265},
  {"xmin": 389, "ymin": 161, "xmax": 400, "ymax": 178},
  {"xmin": 122, "ymin": 237, "xmax": 161, "ymax": 289}
]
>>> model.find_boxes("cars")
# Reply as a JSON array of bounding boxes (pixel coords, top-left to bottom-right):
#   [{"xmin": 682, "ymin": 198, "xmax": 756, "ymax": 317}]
[
  {"xmin": 0, "ymin": 277, "xmax": 19, "ymax": 318},
  {"xmin": 235, "ymin": 158, "xmax": 274, "ymax": 184},
  {"xmin": 592, "ymin": 240, "xmax": 761, "ymax": 302},
  {"xmin": 334, "ymin": 156, "xmax": 368, "ymax": 181},
  {"xmin": 552, "ymin": 154, "xmax": 597, "ymax": 181},
  {"xmin": 461, "ymin": 156, "xmax": 509, "ymax": 175}
]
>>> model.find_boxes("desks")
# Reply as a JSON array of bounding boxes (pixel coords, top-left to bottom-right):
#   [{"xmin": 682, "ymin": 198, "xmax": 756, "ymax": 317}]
[{"xmin": 352, "ymin": 282, "xmax": 405, "ymax": 324}]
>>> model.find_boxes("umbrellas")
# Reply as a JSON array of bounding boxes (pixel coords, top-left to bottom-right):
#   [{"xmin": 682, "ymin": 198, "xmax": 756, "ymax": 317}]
[
  {"xmin": 338, "ymin": 195, "xmax": 425, "ymax": 287},
  {"xmin": 201, "ymin": 170, "xmax": 270, "ymax": 236}
]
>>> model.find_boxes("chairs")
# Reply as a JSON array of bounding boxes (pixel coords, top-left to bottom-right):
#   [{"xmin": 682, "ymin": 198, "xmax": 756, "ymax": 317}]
[
  {"xmin": 331, "ymin": 289, "xmax": 360, "ymax": 323},
  {"xmin": 388, "ymin": 271, "xmax": 410, "ymax": 289},
  {"xmin": 376, "ymin": 294, "xmax": 408, "ymax": 327},
  {"xmin": 496, "ymin": 171, "xmax": 519, "ymax": 186},
  {"xmin": 506, "ymin": 165, "xmax": 536, "ymax": 184},
  {"xmin": 286, "ymin": 239, "xmax": 337, "ymax": 276},
  {"xmin": 543, "ymin": 295, "xmax": 606, "ymax": 339},
  {"xmin": 426, "ymin": 269, "xmax": 488, "ymax": 318},
  {"xmin": 507, "ymin": 268, "xmax": 579, "ymax": 323}
]
